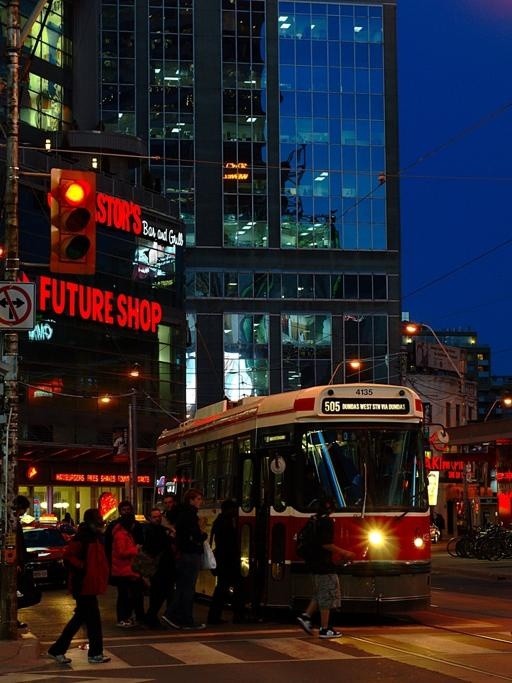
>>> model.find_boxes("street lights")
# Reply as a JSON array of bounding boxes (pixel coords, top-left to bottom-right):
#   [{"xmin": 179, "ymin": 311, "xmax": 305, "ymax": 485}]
[
  {"xmin": 484, "ymin": 396, "xmax": 512, "ymax": 423},
  {"xmin": 98, "ymin": 392, "xmax": 134, "ymax": 515},
  {"xmin": 407, "ymin": 321, "xmax": 467, "ymax": 426},
  {"xmin": 328, "ymin": 359, "xmax": 362, "ymax": 385}
]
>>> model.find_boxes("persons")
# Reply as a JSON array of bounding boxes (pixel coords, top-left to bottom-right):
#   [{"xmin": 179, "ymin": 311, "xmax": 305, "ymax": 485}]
[
  {"xmin": 433, "ymin": 512, "xmax": 446, "ymax": 538},
  {"xmin": 295, "ymin": 501, "xmax": 355, "ymax": 639},
  {"xmin": 103, "ymin": 488, "xmax": 207, "ymax": 629},
  {"xmin": 2, "ymin": 495, "xmax": 42, "ymax": 627},
  {"xmin": 353, "ymin": 443, "xmax": 406, "ymax": 507},
  {"xmin": 113, "ymin": 426, "xmax": 128, "ymax": 455},
  {"xmin": 210, "ymin": 499, "xmax": 255, "ymax": 626},
  {"xmin": 61, "ymin": 512, "xmax": 75, "ymax": 526},
  {"xmin": 47, "ymin": 508, "xmax": 111, "ymax": 663}
]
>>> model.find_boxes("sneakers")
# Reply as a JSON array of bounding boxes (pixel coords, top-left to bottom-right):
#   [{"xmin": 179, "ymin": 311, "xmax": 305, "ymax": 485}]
[
  {"xmin": 88, "ymin": 653, "xmax": 111, "ymax": 663},
  {"xmin": 297, "ymin": 612, "xmax": 313, "ymax": 635},
  {"xmin": 232, "ymin": 619, "xmax": 250, "ymax": 624},
  {"xmin": 115, "ymin": 616, "xmax": 206, "ymax": 630},
  {"xmin": 317, "ymin": 626, "xmax": 343, "ymax": 639},
  {"xmin": 48, "ymin": 648, "xmax": 72, "ymax": 664},
  {"xmin": 207, "ymin": 619, "xmax": 229, "ymax": 628}
]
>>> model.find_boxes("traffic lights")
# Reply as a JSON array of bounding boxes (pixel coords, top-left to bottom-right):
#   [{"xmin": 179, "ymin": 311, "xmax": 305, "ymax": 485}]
[{"xmin": 51, "ymin": 168, "xmax": 96, "ymax": 275}]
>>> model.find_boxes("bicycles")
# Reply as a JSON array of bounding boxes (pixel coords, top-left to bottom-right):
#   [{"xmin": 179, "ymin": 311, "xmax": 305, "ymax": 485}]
[{"xmin": 446, "ymin": 512, "xmax": 512, "ymax": 562}]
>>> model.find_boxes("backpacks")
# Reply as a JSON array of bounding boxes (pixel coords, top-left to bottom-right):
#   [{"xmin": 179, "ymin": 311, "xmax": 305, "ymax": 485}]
[{"xmin": 295, "ymin": 515, "xmax": 331, "ymax": 557}]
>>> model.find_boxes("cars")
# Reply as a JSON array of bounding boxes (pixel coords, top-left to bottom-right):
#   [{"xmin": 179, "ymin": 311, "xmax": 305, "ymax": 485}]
[
  {"xmin": 22, "ymin": 527, "xmax": 69, "ymax": 593},
  {"xmin": 430, "ymin": 522, "xmax": 441, "ymax": 543}
]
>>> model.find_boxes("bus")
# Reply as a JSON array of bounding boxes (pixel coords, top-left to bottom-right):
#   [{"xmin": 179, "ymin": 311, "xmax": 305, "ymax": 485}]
[{"xmin": 153, "ymin": 381, "xmax": 450, "ymax": 611}]
